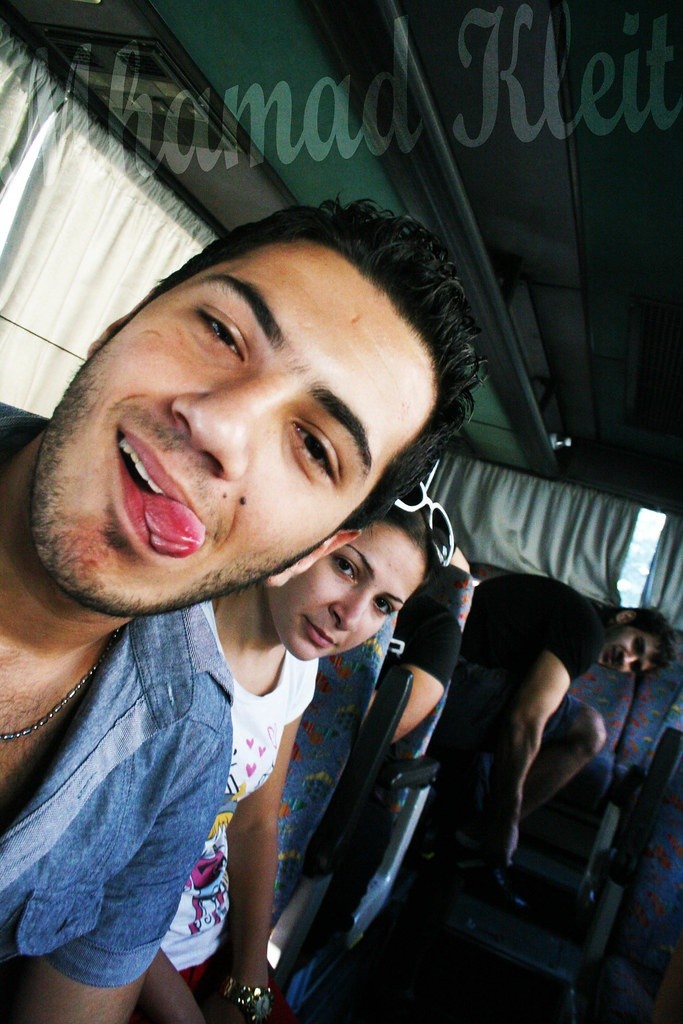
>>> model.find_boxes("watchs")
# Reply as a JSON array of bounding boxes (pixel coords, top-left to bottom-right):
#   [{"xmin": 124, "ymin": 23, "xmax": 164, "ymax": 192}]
[{"xmin": 225, "ymin": 984, "xmax": 275, "ymax": 1024}]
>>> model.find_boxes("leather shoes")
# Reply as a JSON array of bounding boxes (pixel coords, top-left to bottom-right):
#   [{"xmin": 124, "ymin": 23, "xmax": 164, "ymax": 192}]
[{"xmin": 472, "ymin": 864, "xmax": 528, "ymax": 908}]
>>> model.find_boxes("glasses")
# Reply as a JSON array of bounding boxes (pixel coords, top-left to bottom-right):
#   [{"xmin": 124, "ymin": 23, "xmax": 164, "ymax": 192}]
[{"xmin": 395, "ymin": 481, "xmax": 454, "ymax": 567}]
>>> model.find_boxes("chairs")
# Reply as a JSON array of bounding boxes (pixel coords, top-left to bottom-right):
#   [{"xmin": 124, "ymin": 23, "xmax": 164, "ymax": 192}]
[{"xmin": 267, "ymin": 560, "xmax": 683, "ymax": 1024}]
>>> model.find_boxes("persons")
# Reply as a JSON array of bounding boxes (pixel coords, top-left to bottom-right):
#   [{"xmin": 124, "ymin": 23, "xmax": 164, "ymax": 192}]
[
  {"xmin": 0, "ymin": 197, "xmax": 489, "ymax": 1024},
  {"xmin": 131, "ymin": 483, "xmax": 455, "ymax": 1024},
  {"xmin": 356, "ymin": 573, "xmax": 678, "ymax": 1024},
  {"xmin": 359, "ymin": 593, "xmax": 461, "ymax": 745}
]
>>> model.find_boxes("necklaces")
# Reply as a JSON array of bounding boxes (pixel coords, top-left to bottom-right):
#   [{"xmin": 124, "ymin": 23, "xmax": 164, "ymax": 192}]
[{"xmin": 0, "ymin": 627, "xmax": 121, "ymax": 740}]
